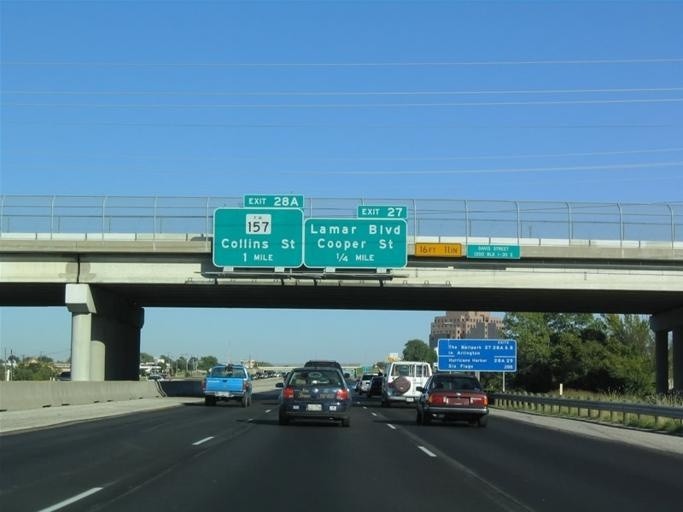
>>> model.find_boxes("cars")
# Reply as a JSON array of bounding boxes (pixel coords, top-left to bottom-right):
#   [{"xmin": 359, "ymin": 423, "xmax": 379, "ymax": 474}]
[
  {"xmin": 276, "ymin": 360, "xmax": 384, "ymax": 427},
  {"xmin": 251, "ymin": 370, "xmax": 286, "ymax": 380},
  {"xmin": 147, "ymin": 374, "xmax": 171, "ymax": 382},
  {"xmin": 59, "ymin": 371, "xmax": 71, "ymax": 381},
  {"xmin": 416, "ymin": 373, "xmax": 489, "ymax": 426}
]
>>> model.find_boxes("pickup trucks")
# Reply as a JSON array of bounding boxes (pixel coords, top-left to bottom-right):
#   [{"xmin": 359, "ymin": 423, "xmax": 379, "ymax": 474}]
[{"xmin": 202, "ymin": 364, "xmax": 253, "ymax": 408}]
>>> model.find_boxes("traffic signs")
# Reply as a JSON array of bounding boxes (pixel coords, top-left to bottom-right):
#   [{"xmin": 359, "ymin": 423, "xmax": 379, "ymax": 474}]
[
  {"xmin": 438, "ymin": 338, "xmax": 517, "ymax": 372},
  {"xmin": 415, "ymin": 243, "xmax": 520, "ymax": 260},
  {"xmin": 212, "ymin": 193, "xmax": 409, "ymax": 269}
]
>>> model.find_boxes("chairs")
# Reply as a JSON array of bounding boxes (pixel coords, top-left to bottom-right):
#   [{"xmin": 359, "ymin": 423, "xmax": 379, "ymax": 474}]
[{"xmin": 294, "ymin": 376, "xmax": 306, "ymax": 386}]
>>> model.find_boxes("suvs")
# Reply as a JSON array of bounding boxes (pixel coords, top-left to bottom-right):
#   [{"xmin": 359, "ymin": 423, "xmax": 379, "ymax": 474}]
[{"xmin": 381, "ymin": 360, "xmax": 432, "ymax": 408}]
